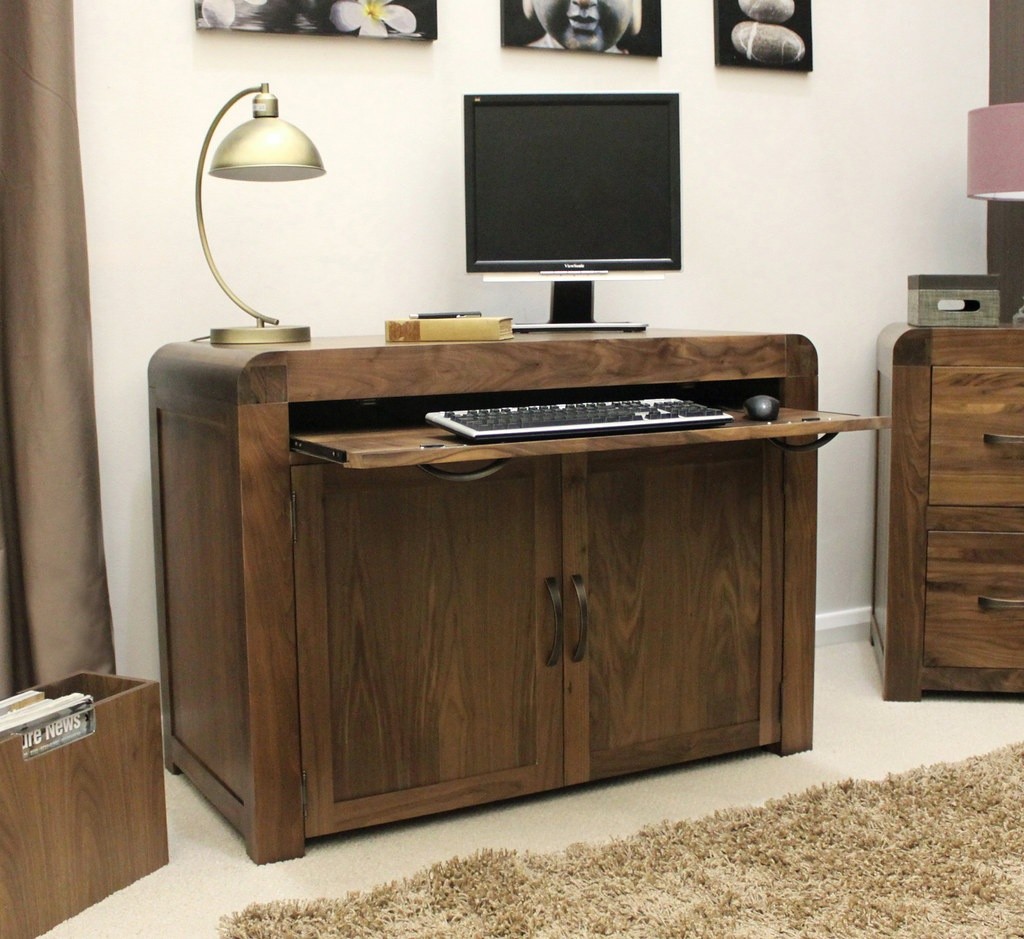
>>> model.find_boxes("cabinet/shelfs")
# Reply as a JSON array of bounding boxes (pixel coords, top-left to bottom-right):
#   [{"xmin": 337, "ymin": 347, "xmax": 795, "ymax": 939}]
[
  {"xmin": 866, "ymin": 321, "xmax": 1024, "ymax": 700},
  {"xmin": 147, "ymin": 332, "xmax": 892, "ymax": 866}
]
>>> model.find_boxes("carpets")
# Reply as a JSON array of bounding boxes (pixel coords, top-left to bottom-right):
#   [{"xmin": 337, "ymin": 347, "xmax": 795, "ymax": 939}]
[{"xmin": 213, "ymin": 744, "xmax": 1024, "ymax": 939}]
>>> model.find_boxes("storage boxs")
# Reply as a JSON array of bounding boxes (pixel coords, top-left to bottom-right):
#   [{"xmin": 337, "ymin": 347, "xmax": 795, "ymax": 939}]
[{"xmin": 0, "ymin": 670, "xmax": 169, "ymax": 939}]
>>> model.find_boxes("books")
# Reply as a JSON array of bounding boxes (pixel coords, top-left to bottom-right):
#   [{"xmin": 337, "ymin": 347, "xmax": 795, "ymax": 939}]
[
  {"xmin": 0, "ymin": 690, "xmax": 93, "ymax": 759},
  {"xmin": 386, "ymin": 317, "xmax": 513, "ymax": 341}
]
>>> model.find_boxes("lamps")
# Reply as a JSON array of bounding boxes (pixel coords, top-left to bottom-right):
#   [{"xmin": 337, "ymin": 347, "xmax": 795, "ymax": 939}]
[
  {"xmin": 968, "ymin": 99, "xmax": 1022, "ymax": 201},
  {"xmin": 196, "ymin": 79, "xmax": 327, "ymax": 344}
]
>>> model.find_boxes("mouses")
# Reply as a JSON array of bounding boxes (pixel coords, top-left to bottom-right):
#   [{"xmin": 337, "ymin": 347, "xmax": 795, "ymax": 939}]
[{"xmin": 743, "ymin": 395, "xmax": 779, "ymax": 421}]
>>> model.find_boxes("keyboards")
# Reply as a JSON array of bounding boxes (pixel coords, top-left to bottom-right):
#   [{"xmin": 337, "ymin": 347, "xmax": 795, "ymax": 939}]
[{"xmin": 425, "ymin": 398, "xmax": 735, "ymax": 442}]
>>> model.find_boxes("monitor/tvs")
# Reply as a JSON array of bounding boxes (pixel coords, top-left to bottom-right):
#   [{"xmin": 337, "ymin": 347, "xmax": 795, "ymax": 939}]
[{"xmin": 464, "ymin": 94, "xmax": 682, "ymax": 331}]
